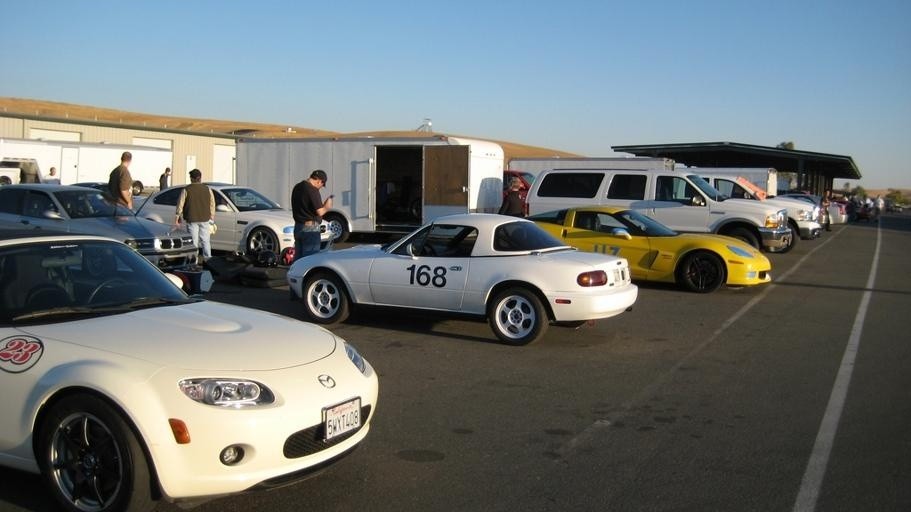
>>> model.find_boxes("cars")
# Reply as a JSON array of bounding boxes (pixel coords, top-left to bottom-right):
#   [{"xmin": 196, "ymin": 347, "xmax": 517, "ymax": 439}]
[
  {"xmin": 774, "ymin": 190, "xmax": 851, "ymax": 227},
  {"xmin": 892, "ymin": 203, "xmax": 903, "ymax": 213}
]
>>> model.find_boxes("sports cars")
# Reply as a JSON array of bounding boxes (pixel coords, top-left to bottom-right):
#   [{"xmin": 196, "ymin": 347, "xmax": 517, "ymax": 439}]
[
  {"xmin": 518, "ymin": 204, "xmax": 775, "ymax": 295},
  {"xmin": 282, "ymin": 212, "xmax": 644, "ymax": 347}
]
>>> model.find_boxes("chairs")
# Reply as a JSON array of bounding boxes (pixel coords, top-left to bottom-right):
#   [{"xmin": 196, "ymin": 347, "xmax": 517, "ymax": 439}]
[
  {"xmin": 1, "ymin": 253, "xmax": 73, "ymax": 311},
  {"xmin": 661, "ymin": 186, "xmax": 671, "ymax": 199},
  {"xmin": 28, "ymin": 193, "xmax": 52, "ymax": 218},
  {"xmin": 585, "ymin": 215, "xmax": 600, "ymax": 231}
]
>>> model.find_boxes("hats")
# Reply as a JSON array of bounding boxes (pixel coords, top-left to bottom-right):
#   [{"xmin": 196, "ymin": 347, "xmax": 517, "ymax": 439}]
[
  {"xmin": 313, "ymin": 170, "xmax": 327, "ymax": 187},
  {"xmin": 189, "ymin": 169, "xmax": 201, "ymax": 179}
]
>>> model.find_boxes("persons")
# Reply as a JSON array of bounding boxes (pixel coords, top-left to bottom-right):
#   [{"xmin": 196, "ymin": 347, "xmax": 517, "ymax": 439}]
[
  {"xmin": 873, "ymin": 194, "xmax": 884, "ymax": 224},
  {"xmin": 289, "ymin": 169, "xmax": 333, "ymax": 299},
  {"xmin": 175, "ymin": 168, "xmax": 215, "ymax": 264},
  {"xmin": 819, "ymin": 189, "xmax": 832, "ymax": 232},
  {"xmin": 863, "ymin": 193, "xmax": 874, "ymax": 222},
  {"xmin": 497, "ymin": 177, "xmax": 525, "ymax": 217},
  {"xmin": 158, "ymin": 167, "xmax": 170, "ymax": 191},
  {"xmin": 108, "ymin": 151, "xmax": 132, "ymax": 209},
  {"xmin": 43, "ymin": 167, "xmax": 59, "ymax": 180}
]
